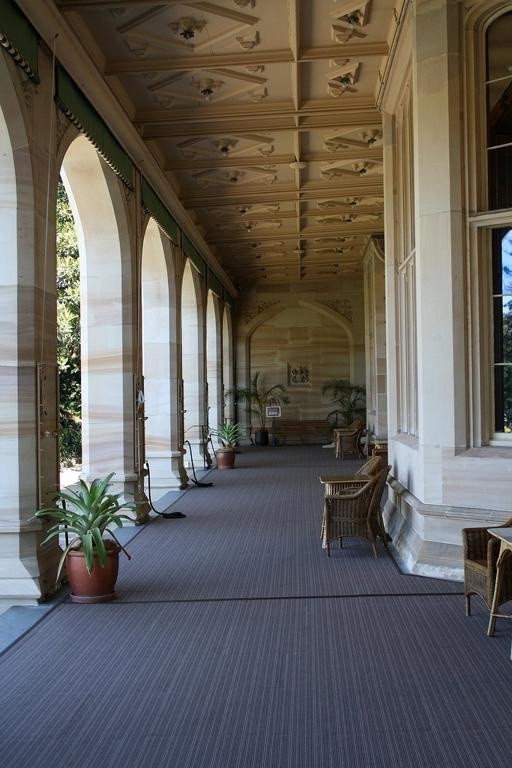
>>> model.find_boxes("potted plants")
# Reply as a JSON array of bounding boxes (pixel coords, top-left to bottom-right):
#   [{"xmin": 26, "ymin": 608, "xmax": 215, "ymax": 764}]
[
  {"xmin": 25, "ymin": 469, "xmax": 149, "ymax": 607},
  {"xmin": 223, "ymin": 368, "xmax": 291, "ymax": 449},
  {"xmin": 210, "ymin": 420, "xmax": 243, "ymax": 468}
]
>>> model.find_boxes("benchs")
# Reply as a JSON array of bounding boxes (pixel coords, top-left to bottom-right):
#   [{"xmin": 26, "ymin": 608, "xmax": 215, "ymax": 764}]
[{"xmin": 266, "ymin": 416, "xmax": 332, "ymax": 446}]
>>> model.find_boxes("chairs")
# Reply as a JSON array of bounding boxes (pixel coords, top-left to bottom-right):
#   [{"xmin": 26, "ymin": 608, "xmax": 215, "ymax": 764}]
[
  {"xmin": 331, "ymin": 416, "xmax": 364, "ymax": 461},
  {"xmin": 459, "ymin": 510, "xmax": 511, "ymax": 639},
  {"xmin": 322, "ymin": 454, "xmax": 391, "ymax": 564}
]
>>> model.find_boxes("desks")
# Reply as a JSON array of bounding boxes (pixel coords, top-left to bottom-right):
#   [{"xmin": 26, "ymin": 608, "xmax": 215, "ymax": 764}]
[
  {"xmin": 482, "ymin": 522, "xmax": 511, "ymax": 637},
  {"xmin": 318, "ymin": 474, "xmax": 387, "ymax": 547}
]
